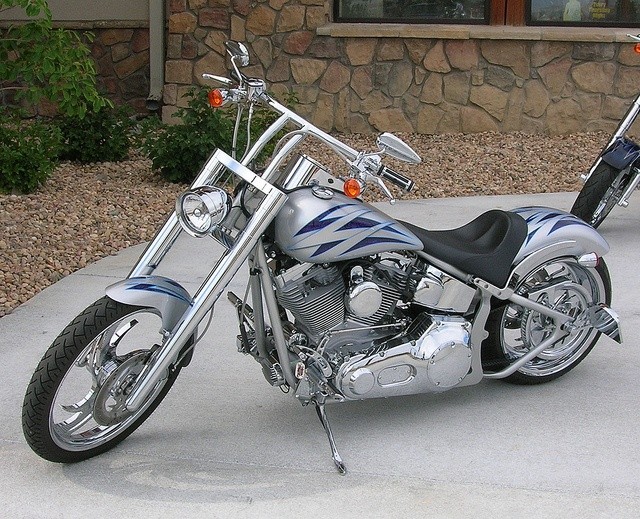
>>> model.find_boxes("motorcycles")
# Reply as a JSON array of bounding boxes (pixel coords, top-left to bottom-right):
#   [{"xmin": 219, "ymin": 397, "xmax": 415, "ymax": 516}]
[
  {"xmin": 570, "ymin": 33, "xmax": 640, "ymax": 229},
  {"xmin": 22, "ymin": 40, "xmax": 623, "ymax": 475}
]
